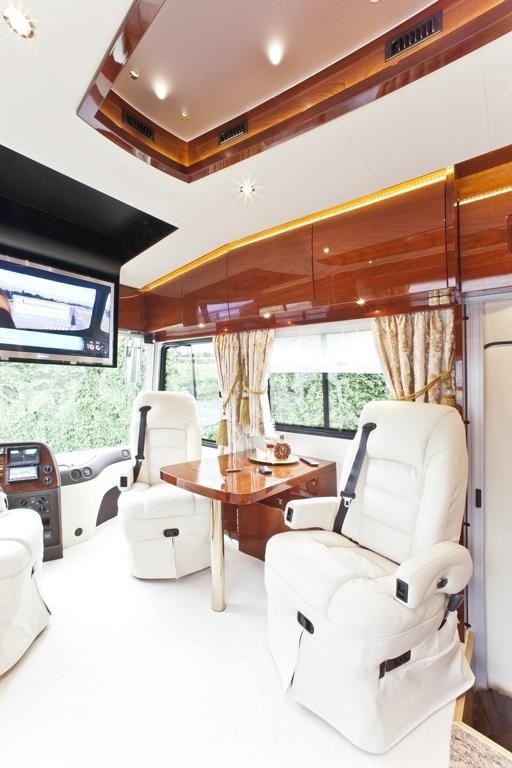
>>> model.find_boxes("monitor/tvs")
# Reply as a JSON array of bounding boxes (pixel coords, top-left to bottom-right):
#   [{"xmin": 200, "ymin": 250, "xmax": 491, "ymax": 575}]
[{"xmin": 0, "ymin": 254, "xmax": 120, "ymax": 368}]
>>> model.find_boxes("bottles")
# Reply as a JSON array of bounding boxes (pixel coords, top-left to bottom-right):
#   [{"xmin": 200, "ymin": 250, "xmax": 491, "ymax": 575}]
[{"xmin": 273, "ymin": 435, "xmax": 291, "ymax": 460}]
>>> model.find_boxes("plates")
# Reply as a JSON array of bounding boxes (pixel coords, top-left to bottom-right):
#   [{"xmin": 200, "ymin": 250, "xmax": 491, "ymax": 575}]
[{"xmin": 248, "ymin": 453, "xmax": 300, "ymax": 465}]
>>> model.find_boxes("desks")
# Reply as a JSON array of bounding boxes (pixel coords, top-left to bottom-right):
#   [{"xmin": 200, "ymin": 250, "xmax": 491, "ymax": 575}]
[{"xmin": 160, "ymin": 444, "xmax": 336, "ymax": 611}]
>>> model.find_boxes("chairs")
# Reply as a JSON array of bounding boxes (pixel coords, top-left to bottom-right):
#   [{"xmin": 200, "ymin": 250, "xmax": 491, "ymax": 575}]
[
  {"xmin": 0, "ymin": 490, "xmax": 51, "ymax": 677},
  {"xmin": 117, "ymin": 390, "xmax": 213, "ymax": 581},
  {"xmin": 265, "ymin": 398, "xmax": 477, "ymax": 755}
]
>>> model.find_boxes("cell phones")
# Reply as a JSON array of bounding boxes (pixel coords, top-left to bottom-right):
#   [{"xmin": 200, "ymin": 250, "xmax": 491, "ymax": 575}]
[{"xmin": 259, "ymin": 465, "xmax": 273, "ymax": 474}]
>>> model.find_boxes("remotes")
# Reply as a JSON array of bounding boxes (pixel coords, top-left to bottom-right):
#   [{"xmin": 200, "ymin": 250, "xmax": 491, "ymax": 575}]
[{"xmin": 301, "ymin": 456, "xmax": 320, "ymax": 466}]
